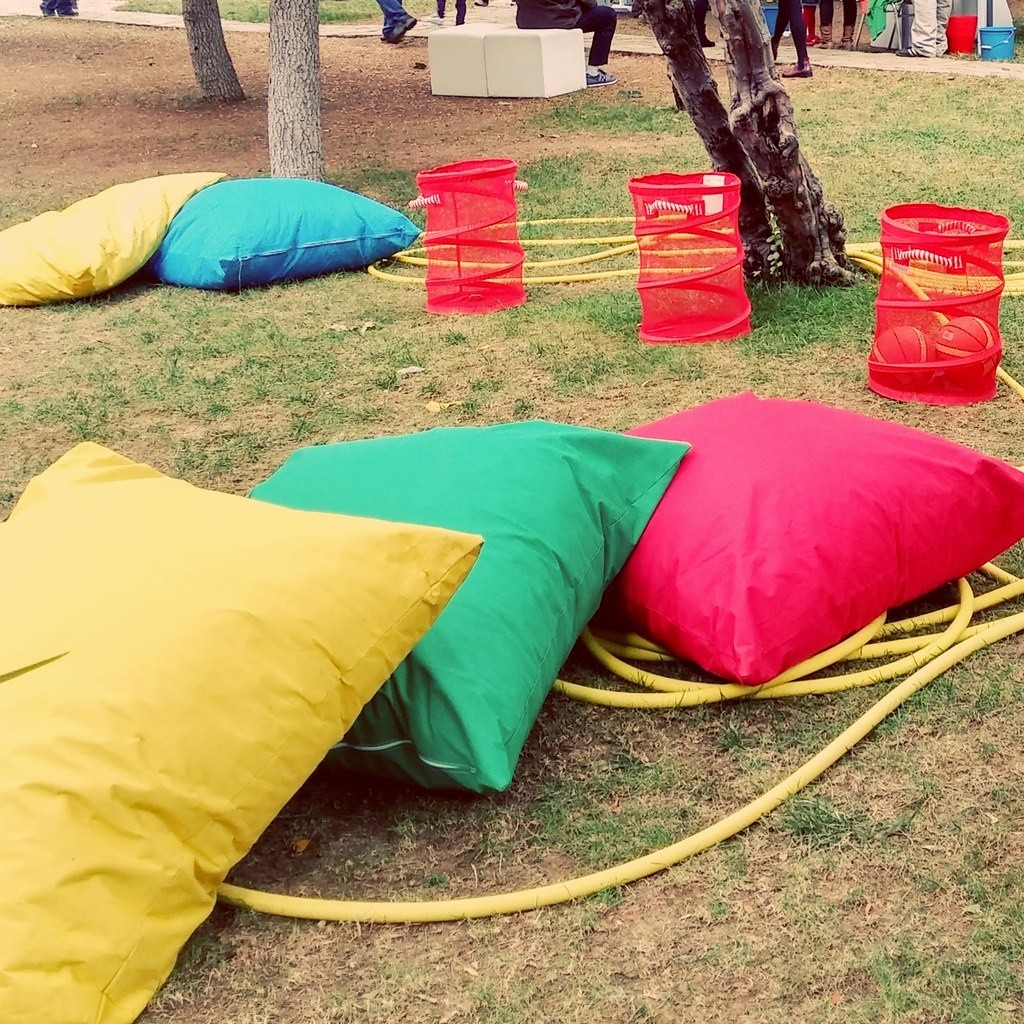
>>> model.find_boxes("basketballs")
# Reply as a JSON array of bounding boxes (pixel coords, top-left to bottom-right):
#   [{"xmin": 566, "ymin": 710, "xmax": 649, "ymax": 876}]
[
  {"xmin": 932, "ymin": 315, "xmax": 1002, "ymax": 381},
  {"xmin": 872, "ymin": 325, "xmax": 936, "ymax": 389}
]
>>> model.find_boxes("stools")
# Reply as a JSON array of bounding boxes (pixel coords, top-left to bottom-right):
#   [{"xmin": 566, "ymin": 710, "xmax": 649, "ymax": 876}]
[{"xmin": 427, "ymin": 23, "xmax": 587, "ymax": 98}]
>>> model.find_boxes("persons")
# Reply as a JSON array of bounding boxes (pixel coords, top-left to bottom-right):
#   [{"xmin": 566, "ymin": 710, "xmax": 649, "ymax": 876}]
[
  {"xmin": 40, "ymin": 0, "xmax": 79, "ymax": 17},
  {"xmin": 375, "ymin": 0, "xmax": 489, "ymax": 43},
  {"xmin": 627, "ymin": 0, "xmax": 953, "ymax": 78},
  {"xmin": 513, "ymin": 0, "xmax": 618, "ymax": 87}
]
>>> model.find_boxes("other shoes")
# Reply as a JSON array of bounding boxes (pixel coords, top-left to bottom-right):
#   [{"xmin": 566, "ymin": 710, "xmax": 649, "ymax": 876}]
[
  {"xmin": 380, "ymin": 34, "xmax": 388, "ymax": 41},
  {"xmin": 701, "ymin": 41, "xmax": 715, "ymax": 47},
  {"xmin": 388, "ymin": 17, "xmax": 417, "ymax": 43},
  {"xmin": 474, "ymin": 0, "xmax": 488, "ymax": 7},
  {"xmin": 782, "ymin": 57, "xmax": 815, "ymax": 78}
]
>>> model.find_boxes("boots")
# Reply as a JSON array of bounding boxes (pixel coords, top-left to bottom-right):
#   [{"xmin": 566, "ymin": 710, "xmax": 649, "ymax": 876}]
[
  {"xmin": 813, "ymin": 23, "xmax": 835, "ymax": 48},
  {"xmin": 831, "ymin": 25, "xmax": 855, "ymax": 51}
]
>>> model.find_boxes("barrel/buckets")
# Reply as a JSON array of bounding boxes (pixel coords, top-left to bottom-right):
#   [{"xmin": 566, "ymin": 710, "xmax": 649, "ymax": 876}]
[
  {"xmin": 946, "ymin": 15, "xmax": 978, "ymax": 53},
  {"xmin": 980, "ymin": 27, "xmax": 1017, "ymax": 62}
]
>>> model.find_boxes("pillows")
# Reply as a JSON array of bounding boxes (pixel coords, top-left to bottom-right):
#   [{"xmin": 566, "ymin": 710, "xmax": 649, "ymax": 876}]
[
  {"xmin": 591, "ymin": 391, "xmax": 1022, "ymax": 686},
  {"xmin": 0, "ymin": 441, "xmax": 483, "ymax": 1024},
  {"xmin": 0, "ymin": 172, "xmax": 423, "ymax": 305},
  {"xmin": 251, "ymin": 421, "xmax": 692, "ymax": 794}
]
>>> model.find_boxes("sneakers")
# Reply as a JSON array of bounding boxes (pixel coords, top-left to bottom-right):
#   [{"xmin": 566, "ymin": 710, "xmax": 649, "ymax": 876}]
[
  {"xmin": 428, "ymin": 16, "xmax": 444, "ymax": 26},
  {"xmin": 896, "ymin": 46, "xmax": 928, "ymax": 57},
  {"xmin": 586, "ymin": 67, "xmax": 618, "ymax": 88}
]
